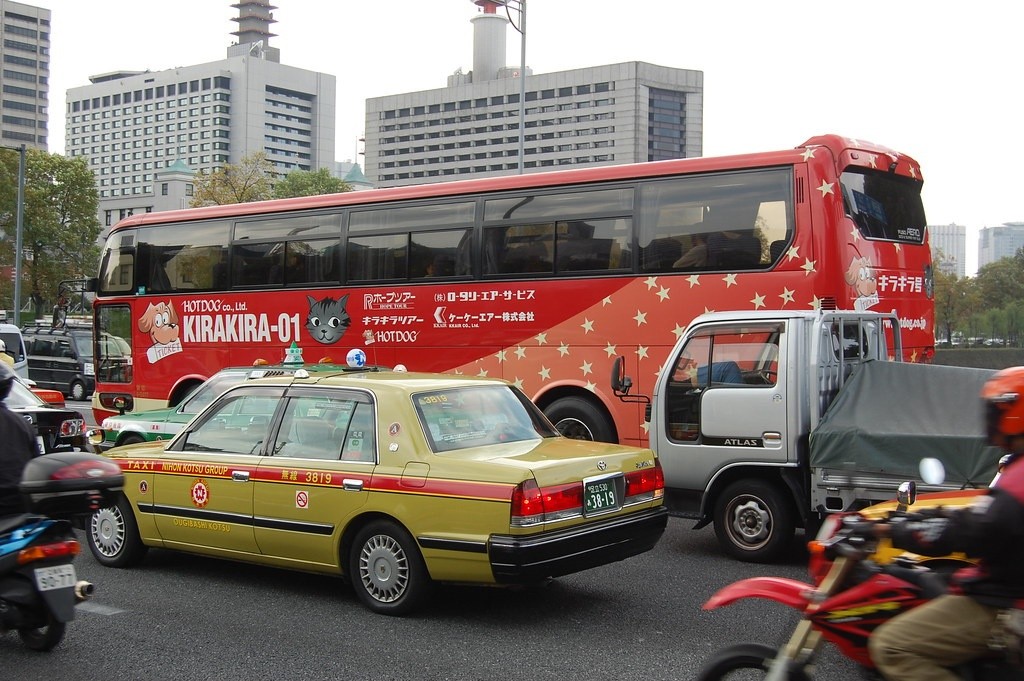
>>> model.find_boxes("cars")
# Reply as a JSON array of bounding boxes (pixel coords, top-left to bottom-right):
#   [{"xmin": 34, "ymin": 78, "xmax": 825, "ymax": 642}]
[
  {"xmin": 84, "ymin": 364, "xmax": 667, "ymax": 617},
  {"xmin": 1, "ymin": 376, "xmax": 86, "ymax": 456},
  {"xmin": 93, "ymin": 364, "xmax": 488, "ymax": 454},
  {"xmin": 809, "ymin": 488, "xmax": 987, "ymax": 588}
]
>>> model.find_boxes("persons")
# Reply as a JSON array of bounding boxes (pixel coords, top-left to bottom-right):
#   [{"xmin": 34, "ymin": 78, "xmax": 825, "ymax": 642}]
[
  {"xmin": 672, "ymin": 222, "xmax": 709, "ymax": 268},
  {"xmin": 269, "ymin": 253, "xmax": 302, "ymax": 288},
  {"xmin": 869, "ymin": 366, "xmax": 1024, "ymax": 681},
  {"xmin": 0, "ymin": 361, "xmax": 39, "ymax": 521},
  {"xmin": 52, "ymin": 294, "xmax": 69, "ymax": 331}
]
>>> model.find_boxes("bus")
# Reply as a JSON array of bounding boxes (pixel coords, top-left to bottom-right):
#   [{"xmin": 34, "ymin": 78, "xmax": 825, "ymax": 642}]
[{"xmin": 53, "ymin": 135, "xmax": 936, "ymax": 448}]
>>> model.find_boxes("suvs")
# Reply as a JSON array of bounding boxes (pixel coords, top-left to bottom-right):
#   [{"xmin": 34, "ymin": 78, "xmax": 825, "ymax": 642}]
[{"xmin": 21, "ymin": 323, "xmax": 129, "ymax": 402}]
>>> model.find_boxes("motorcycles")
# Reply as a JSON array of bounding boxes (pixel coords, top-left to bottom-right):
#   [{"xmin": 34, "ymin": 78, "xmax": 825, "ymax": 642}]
[
  {"xmin": 696, "ymin": 482, "xmax": 1024, "ymax": 681},
  {"xmin": 0, "ymin": 452, "xmax": 127, "ymax": 653}
]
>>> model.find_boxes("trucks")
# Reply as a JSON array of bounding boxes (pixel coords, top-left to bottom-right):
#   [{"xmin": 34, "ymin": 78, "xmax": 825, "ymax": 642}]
[{"xmin": 611, "ymin": 308, "xmax": 1010, "ymax": 563}]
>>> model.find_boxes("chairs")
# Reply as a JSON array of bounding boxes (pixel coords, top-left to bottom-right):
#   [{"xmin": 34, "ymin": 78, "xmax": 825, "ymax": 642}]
[{"xmin": 279, "ymin": 418, "xmax": 338, "ymax": 459}]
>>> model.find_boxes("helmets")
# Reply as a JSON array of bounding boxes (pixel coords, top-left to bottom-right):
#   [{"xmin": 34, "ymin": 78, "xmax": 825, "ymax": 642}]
[
  {"xmin": 980, "ymin": 366, "xmax": 1024, "ymax": 436},
  {"xmin": 0, "ymin": 339, "xmax": 5, "ymax": 351}
]
